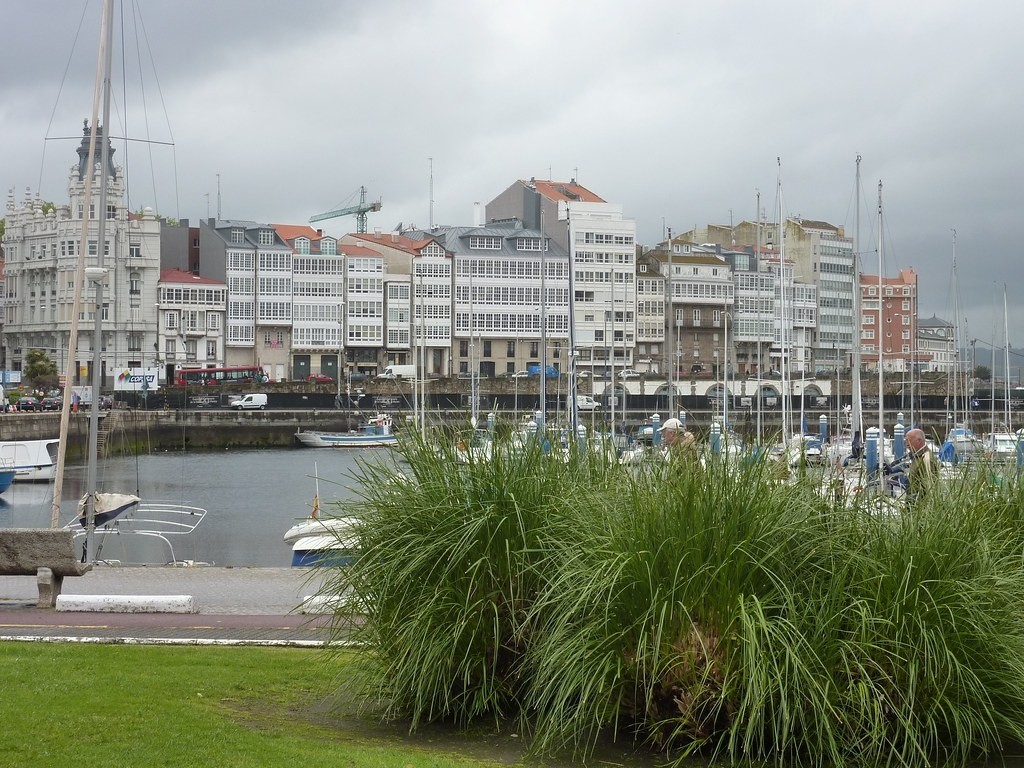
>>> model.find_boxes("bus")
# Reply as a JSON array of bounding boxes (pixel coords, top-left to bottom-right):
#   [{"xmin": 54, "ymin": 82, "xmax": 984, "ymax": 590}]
[{"xmin": 177, "ymin": 366, "xmax": 270, "ymax": 387}]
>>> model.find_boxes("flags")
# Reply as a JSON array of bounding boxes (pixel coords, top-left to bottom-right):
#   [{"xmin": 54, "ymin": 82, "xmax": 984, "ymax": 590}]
[{"xmin": 311, "ymin": 495, "xmax": 318, "ymax": 517}]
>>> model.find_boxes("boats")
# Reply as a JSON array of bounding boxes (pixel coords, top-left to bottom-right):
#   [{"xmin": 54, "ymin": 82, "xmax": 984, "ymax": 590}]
[{"xmin": 0, "ymin": 440, "xmax": 62, "ymax": 482}]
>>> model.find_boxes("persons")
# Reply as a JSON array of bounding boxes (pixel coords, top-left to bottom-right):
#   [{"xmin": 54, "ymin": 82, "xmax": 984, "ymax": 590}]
[
  {"xmin": 904, "ymin": 429, "xmax": 940, "ymax": 511},
  {"xmin": 194, "ymin": 375, "xmax": 217, "ymax": 385},
  {"xmin": 656, "ymin": 418, "xmax": 705, "ymax": 481},
  {"xmin": 242, "ymin": 371, "xmax": 269, "ymax": 383}
]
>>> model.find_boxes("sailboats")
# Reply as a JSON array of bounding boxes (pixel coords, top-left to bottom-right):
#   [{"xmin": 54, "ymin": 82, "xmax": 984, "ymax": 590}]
[
  {"xmin": 283, "ymin": 155, "xmax": 1024, "ymax": 566},
  {"xmin": 42, "ymin": 0, "xmax": 214, "ymax": 567}
]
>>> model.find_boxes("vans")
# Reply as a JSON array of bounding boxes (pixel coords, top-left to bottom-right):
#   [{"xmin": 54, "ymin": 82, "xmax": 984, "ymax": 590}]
[
  {"xmin": 231, "ymin": 393, "xmax": 268, "ymax": 410},
  {"xmin": 376, "ymin": 364, "xmax": 416, "ymax": 379},
  {"xmin": 566, "ymin": 395, "xmax": 602, "ymax": 411},
  {"xmin": 528, "ymin": 365, "xmax": 559, "ymax": 379}
]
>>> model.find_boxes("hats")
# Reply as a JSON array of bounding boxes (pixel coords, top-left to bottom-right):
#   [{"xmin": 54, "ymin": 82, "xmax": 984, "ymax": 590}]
[{"xmin": 656, "ymin": 418, "xmax": 684, "ymax": 433}]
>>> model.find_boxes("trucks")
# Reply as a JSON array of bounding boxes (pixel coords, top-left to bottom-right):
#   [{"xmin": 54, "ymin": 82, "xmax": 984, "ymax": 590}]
[{"xmin": 55, "ymin": 385, "xmax": 92, "ymax": 413}]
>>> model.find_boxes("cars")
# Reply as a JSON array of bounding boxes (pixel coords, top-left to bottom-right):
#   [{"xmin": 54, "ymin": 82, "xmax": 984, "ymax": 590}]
[
  {"xmin": 343, "ymin": 372, "xmax": 368, "ymax": 380},
  {"xmin": 498, "ymin": 370, "xmax": 529, "ymax": 378},
  {"xmin": 427, "ymin": 372, "xmax": 445, "ymax": 378},
  {"xmin": 16, "ymin": 396, "xmax": 44, "ymax": 412},
  {"xmin": 754, "ymin": 369, "xmax": 782, "ymax": 377},
  {"xmin": 576, "ymin": 369, "xmax": 602, "ymax": 377},
  {"xmin": 97, "ymin": 396, "xmax": 113, "ymax": 409},
  {"xmin": 617, "ymin": 368, "xmax": 640, "ymax": 378},
  {"xmin": 306, "ymin": 373, "xmax": 333, "ymax": 381},
  {"xmin": 40, "ymin": 398, "xmax": 63, "ymax": 410}
]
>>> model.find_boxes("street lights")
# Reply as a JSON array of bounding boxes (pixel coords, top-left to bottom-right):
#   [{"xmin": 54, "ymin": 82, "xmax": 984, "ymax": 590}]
[
  {"xmin": 450, "ymin": 297, "xmax": 459, "ymax": 376},
  {"xmin": 337, "ymin": 302, "xmax": 345, "ymax": 408},
  {"xmin": 154, "ymin": 303, "xmax": 160, "ymax": 385},
  {"xmin": 720, "ymin": 310, "xmax": 745, "ymax": 379}
]
